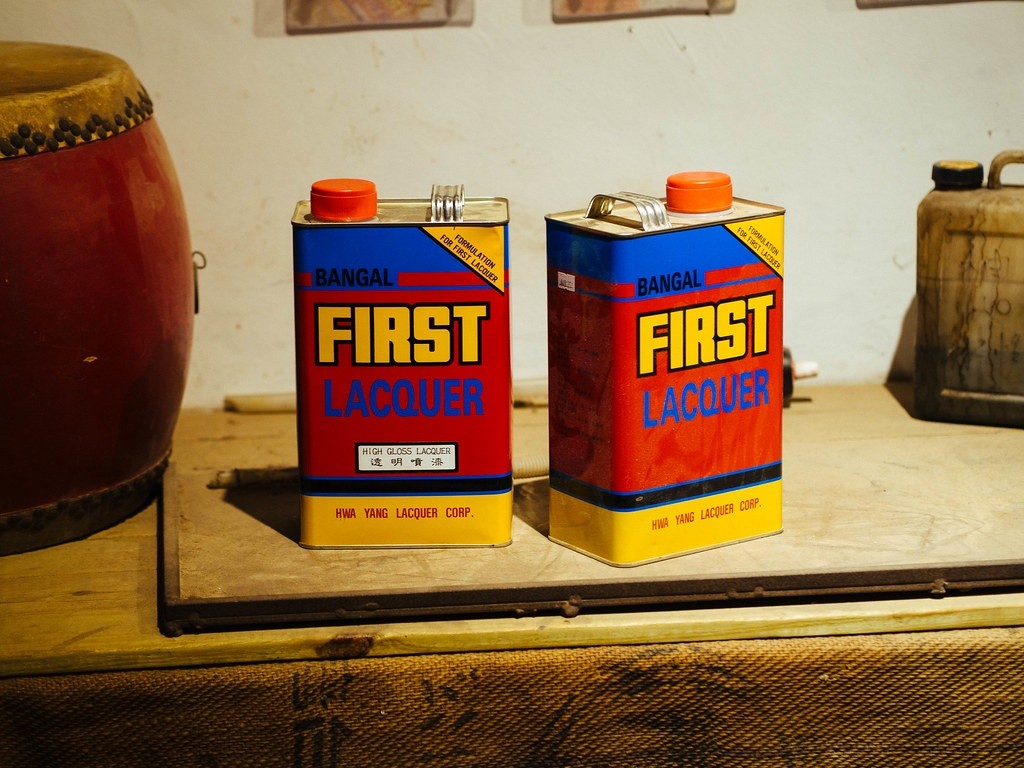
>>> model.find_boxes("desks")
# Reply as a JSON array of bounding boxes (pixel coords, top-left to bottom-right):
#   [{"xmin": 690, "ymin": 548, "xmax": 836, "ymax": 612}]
[{"xmin": 0, "ymin": 381, "xmax": 1024, "ymax": 768}]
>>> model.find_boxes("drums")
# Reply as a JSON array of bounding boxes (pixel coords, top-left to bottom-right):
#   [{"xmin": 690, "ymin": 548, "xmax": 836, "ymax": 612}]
[{"xmin": 0, "ymin": 35, "xmax": 199, "ymax": 555}]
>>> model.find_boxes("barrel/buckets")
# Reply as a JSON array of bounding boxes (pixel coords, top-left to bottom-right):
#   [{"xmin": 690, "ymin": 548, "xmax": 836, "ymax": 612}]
[
  {"xmin": 543, "ymin": 172, "xmax": 790, "ymax": 568},
  {"xmin": 288, "ymin": 177, "xmax": 514, "ymax": 549},
  {"xmin": 915, "ymin": 149, "xmax": 1023, "ymax": 431}
]
